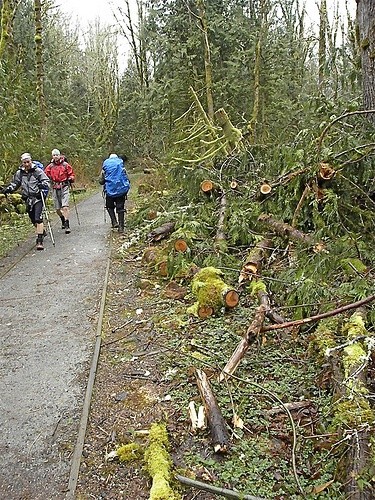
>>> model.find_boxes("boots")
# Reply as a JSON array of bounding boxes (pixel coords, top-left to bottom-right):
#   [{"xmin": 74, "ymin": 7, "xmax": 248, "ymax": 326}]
[
  {"xmin": 118, "ymin": 212, "xmax": 124, "ymax": 232},
  {"xmin": 60, "ymin": 216, "xmax": 65, "ymax": 228},
  {"xmin": 36, "ymin": 230, "xmax": 47, "ymax": 242},
  {"xmin": 108, "ymin": 208, "xmax": 119, "ymax": 228},
  {"xmin": 37, "ymin": 234, "xmax": 44, "ymax": 249},
  {"xmin": 65, "ymin": 220, "xmax": 70, "ymax": 234}
]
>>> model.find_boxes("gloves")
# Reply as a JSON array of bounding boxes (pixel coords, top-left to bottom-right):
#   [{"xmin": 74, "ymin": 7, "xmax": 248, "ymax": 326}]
[
  {"xmin": 38, "ymin": 185, "xmax": 45, "ymax": 189},
  {"xmin": 1, "ymin": 187, "xmax": 13, "ymax": 194}
]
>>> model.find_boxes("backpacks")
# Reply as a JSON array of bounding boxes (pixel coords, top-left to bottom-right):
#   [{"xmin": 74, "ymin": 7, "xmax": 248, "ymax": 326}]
[
  {"xmin": 51, "ymin": 156, "xmax": 68, "ymax": 163},
  {"xmin": 102, "ymin": 158, "xmax": 130, "ymax": 198},
  {"xmin": 19, "ymin": 161, "xmax": 43, "ymax": 170}
]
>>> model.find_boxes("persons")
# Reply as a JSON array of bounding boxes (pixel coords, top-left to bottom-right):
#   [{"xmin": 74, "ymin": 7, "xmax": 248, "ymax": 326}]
[
  {"xmin": 45, "ymin": 149, "xmax": 75, "ymax": 233},
  {"xmin": 4, "ymin": 153, "xmax": 50, "ymax": 250},
  {"xmin": 99, "ymin": 154, "xmax": 125, "ymax": 233}
]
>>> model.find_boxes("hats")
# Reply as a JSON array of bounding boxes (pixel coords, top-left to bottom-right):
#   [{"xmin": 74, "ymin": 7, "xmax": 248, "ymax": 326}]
[
  {"xmin": 110, "ymin": 154, "xmax": 118, "ymax": 158},
  {"xmin": 21, "ymin": 153, "xmax": 31, "ymax": 161},
  {"xmin": 52, "ymin": 149, "xmax": 60, "ymax": 156}
]
